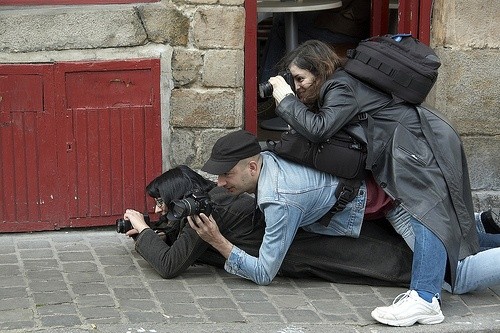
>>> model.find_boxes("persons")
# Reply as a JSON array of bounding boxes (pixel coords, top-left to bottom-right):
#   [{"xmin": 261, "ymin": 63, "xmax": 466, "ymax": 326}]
[
  {"xmin": 268, "ymin": 40, "xmax": 500, "ymax": 326},
  {"xmin": 124, "ymin": 165, "xmax": 500, "ymax": 288},
  {"xmin": 187, "ymin": 130, "xmax": 500, "ymax": 294}
]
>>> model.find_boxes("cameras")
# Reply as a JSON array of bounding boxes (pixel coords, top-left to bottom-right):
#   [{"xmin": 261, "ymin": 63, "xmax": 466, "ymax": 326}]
[
  {"xmin": 258, "ymin": 71, "xmax": 295, "ymax": 98},
  {"xmin": 167, "ymin": 189, "xmax": 212, "ymax": 218},
  {"xmin": 115, "ymin": 214, "xmax": 151, "ymax": 234}
]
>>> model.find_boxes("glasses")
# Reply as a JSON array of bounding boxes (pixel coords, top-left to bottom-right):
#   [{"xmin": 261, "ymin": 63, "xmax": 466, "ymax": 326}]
[{"xmin": 156, "ymin": 200, "xmax": 164, "ymax": 208}]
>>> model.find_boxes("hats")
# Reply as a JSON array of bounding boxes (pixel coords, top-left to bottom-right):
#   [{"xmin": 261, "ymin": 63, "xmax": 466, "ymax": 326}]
[{"xmin": 201, "ymin": 130, "xmax": 261, "ymax": 175}]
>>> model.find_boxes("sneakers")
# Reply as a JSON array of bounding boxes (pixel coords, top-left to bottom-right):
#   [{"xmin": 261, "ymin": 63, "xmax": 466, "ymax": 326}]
[{"xmin": 371, "ymin": 289, "xmax": 445, "ymax": 327}]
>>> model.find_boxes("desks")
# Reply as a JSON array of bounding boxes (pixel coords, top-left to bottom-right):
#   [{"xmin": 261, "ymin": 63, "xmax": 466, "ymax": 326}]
[{"xmin": 257, "ymin": 0, "xmax": 343, "ymax": 131}]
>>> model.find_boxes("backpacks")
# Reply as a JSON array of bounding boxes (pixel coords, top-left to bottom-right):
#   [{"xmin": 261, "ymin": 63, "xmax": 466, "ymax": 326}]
[
  {"xmin": 335, "ymin": 34, "xmax": 441, "ymax": 126},
  {"xmin": 251, "ymin": 130, "xmax": 369, "ymax": 228}
]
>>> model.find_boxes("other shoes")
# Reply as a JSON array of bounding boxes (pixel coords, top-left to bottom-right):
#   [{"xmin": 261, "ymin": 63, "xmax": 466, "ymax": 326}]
[{"xmin": 480, "ymin": 208, "xmax": 500, "ymax": 234}]
